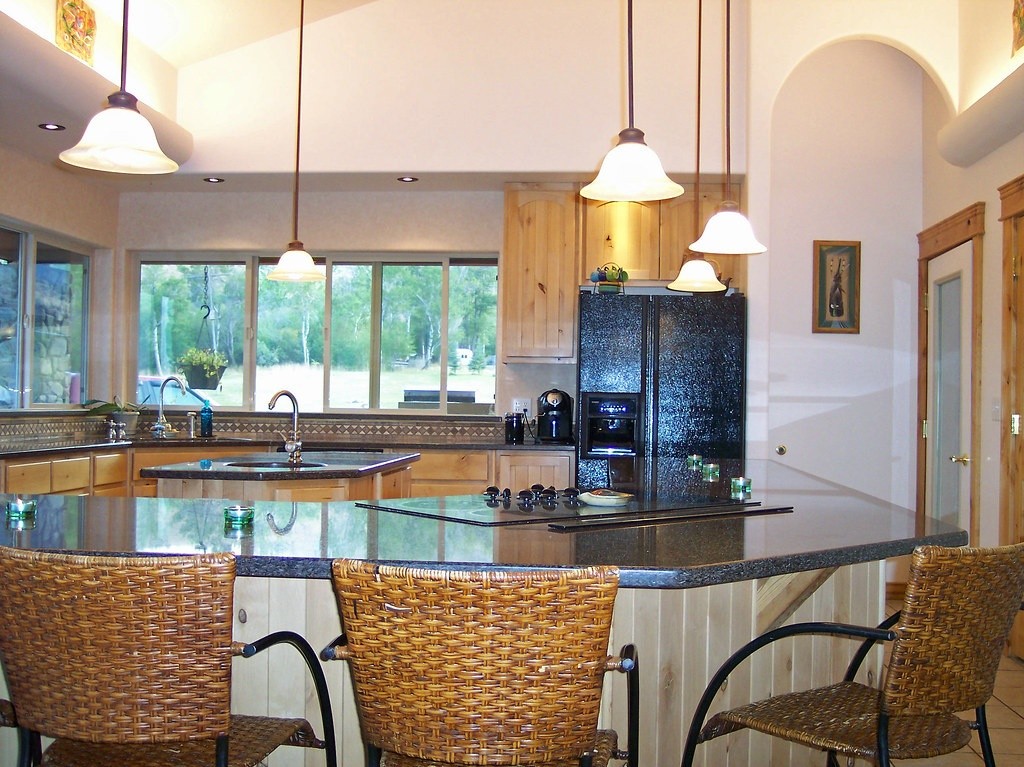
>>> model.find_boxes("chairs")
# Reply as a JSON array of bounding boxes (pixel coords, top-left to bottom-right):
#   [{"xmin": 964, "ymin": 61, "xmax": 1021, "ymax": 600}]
[
  {"xmin": 321, "ymin": 558, "xmax": 643, "ymax": 766},
  {"xmin": 679, "ymin": 543, "xmax": 1024, "ymax": 767},
  {"xmin": 0, "ymin": 540, "xmax": 337, "ymax": 767}
]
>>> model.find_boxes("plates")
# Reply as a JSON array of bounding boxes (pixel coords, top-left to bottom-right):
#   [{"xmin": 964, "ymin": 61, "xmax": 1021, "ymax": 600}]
[
  {"xmin": 577, "ymin": 505, "xmax": 629, "ymax": 520},
  {"xmin": 577, "ymin": 491, "xmax": 635, "ymax": 507}
]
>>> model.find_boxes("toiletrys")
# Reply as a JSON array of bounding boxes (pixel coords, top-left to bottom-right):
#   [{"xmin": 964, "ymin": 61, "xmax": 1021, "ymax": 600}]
[
  {"xmin": 200, "ymin": 459, "xmax": 212, "ymax": 470},
  {"xmin": 200, "ymin": 399, "xmax": 213, "ymax": 436}
]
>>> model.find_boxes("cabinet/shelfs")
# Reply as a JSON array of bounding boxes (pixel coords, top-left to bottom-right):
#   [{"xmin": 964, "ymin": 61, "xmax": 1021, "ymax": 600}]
[
  {"xmin": 376, "ymin": 464, "xmax": 412, "ymax": 499},
  {"xmin": 5, "ymin": 452, "xmax": 129, "ymax": 501},
  {"xmin": 391, "ymin": 448, "xmax": 487, "ymax": 499},
  {"xmin": 503, "ymin": 187, "xmax": 586, "ymax": 366},
  {"xmin": 496, "ymin": 450, "xmax": 569, "ymax": 494},
  {"xmin": 584, "ymin": 181, "xmax": 740, "ymax": 281}
]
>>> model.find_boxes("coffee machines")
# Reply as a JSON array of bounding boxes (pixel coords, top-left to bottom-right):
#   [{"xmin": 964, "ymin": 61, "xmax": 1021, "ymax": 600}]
[{"xmin": 534, "ymin": 389, "xmax": 574, "ymax": 445}]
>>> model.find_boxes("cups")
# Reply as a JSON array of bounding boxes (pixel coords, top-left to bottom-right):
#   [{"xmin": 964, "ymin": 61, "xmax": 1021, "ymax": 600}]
[{"xmin": 505, "ymin": 413, "xmax": 524, "ymax": 442}]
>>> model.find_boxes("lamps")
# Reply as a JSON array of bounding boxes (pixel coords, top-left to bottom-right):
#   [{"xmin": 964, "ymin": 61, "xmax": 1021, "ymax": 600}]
[
  {"xmin": 687, "ymin": 1, "xmax": 767, "ymax": 254},
  {"xmin": 59, "ymin": 1, "xmax": 179, "ymax": 173},
  {"xmin": 579, "ymin": 0, "xmax": 684, "ymax": 202},
  {"xmin": 667, "ymin": 0, "xmax": 727, "ymax": 294},
  {"xmin": 266, "ymin": 0, "xmax": 327, "ymax": 281}
]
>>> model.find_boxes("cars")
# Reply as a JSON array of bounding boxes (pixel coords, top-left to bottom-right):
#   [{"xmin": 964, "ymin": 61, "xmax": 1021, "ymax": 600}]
[{"xmin": 137, "ymin": 376, "xmax": 216, "ymax": 407}]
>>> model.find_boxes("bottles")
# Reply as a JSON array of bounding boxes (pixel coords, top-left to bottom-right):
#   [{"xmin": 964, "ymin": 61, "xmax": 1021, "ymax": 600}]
[{"xmin": 200, "ymin": 400, "xmax": 213, "ymax": 437}]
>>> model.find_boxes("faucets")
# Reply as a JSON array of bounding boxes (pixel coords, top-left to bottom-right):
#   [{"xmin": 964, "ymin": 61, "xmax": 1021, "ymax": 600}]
[
  {"xmin": 158, "ymin": 375, "xmax": 186, "ymax": 423},
  {"xmin": 268, "ymin": 390, "xmax": 303, "ymax": 462},
  {"xmin": 266, "ymin": 502, "xmax": 297, "ymax": 536}
]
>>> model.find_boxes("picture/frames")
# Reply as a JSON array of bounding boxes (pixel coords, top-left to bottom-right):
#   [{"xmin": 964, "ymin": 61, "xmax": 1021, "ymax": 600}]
[{"xmin": 811, "ymin": 240, "xmax": 862, "ymax": 334}]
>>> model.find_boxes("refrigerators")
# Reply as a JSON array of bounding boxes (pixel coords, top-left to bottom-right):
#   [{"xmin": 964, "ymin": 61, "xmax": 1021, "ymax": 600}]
[{"xmin": 576, "ymin": 286, "xmax": 747, "ymax": 457}]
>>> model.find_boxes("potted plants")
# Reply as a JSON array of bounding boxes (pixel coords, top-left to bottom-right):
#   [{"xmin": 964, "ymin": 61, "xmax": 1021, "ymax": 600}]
[
  {"xmin": 177, "ymin": 347, "xmax": 228, "ymax": 391},
  {"xmin": 82, "ymin": 396, "xmax": 151, "ymax": 436}
]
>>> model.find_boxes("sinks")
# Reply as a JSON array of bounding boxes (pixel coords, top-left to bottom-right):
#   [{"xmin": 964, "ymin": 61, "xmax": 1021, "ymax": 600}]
[
  {"xmin": 224, "ymin": 461, "xmax": 329, "ymax": 468},
  {"xmin": 119, "ymin": 438, "xmax": 208, "ymax": 440}
]
